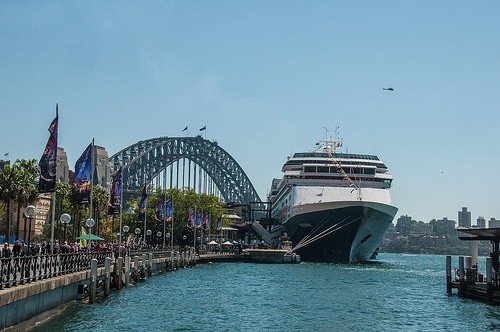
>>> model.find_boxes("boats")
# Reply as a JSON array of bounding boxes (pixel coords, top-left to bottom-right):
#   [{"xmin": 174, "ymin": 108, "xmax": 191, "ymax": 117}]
[{"xmin": 266, "ymin": 126, "xmax": 400, "ymax": 266}]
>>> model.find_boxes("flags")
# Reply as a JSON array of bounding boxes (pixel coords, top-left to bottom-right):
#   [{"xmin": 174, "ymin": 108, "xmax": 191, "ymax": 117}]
[
  {"xmin": 155, "ymin": 196, "xmax": 172, "ymax": 223},
  {"xmin": 217, "ymin": 216, "xmax": 222, "ymax": 230},
  {"xmin": 187, "ymin": 209, "xmax": 210, "ymax": 231},
  {"xmin": 108, "ymin": 169, "xmax": 121, "ymax": 214},
  {"xmin": 72, "ymin": 143, "xmax": 91, "ymax": 205},
  {"xmin": 139, "ymin": 185, "xmax": 148, "ymax": 209},
  {"xmin": 200, "ymin": 126, "xmax": 206, "ymax": 131},
  {"xmin": 182, "ymin": 127, "xmax": 187, "ymax": 132},
  {"xmin": 38, "ymin": 118, "xmax": 56, "ymax": 193}
]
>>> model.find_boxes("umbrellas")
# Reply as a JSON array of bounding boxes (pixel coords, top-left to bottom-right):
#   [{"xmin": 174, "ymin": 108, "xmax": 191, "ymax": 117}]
[
  {"xmin": 206, "ymin": 240, "xmax": 238, "ymax": 245},
  {"xmin": 75, "ymin": 233, "xmax": 104, "ymax": 240}
]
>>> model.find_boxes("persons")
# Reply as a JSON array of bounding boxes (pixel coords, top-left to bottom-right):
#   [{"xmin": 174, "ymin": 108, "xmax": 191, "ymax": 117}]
[{"xmin": 0, "ymin": 240, "xmax": 125, "ymax": 265}]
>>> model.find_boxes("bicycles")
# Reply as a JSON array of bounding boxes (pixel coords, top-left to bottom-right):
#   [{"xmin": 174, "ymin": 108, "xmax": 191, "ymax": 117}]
[{"xmin": 1, "ymin": 252, "xmax": 22, "ymax": 276}]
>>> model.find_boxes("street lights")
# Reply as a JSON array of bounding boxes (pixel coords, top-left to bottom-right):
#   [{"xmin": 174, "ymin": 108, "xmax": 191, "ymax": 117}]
[
  {"xmin": 146, "ymin": 230, "xmax": 152, "ymax": 252},
  {"xmin": 166, "ymin": 233, "xmax": 171, "ymax": 248},
  {"xmin": 123, "ymin": 226, "xmax": 130, "ymax": 262},
  {"xmin": 156, "ymin": 231, "xmax": 162, "ymax": 251},
  {"xmin": 182, "ymin": 235, "xmax": 187, "ymax": 248},
  {"xmin": 86, "ymin": 218, "xmax": 95, "ymax": 258},
  {"xmin": 25, "ymin": 205, "xmax": 38, "ymax": 252},
  {"xmin": 134, "ymin": 228, "xmax": 141, "ymax": 250},
  {"xmin": 60, "ymin": 213, "xmax": 71, "ymax": 275}
]
